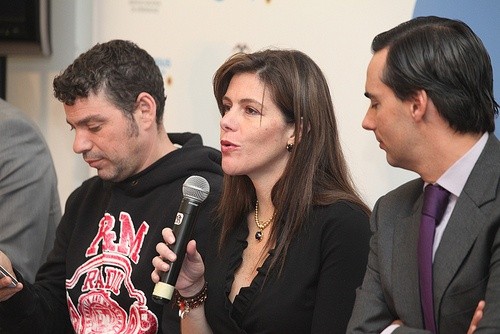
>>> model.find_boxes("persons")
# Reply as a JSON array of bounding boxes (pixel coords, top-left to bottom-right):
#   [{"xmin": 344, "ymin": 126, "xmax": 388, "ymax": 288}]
[
  {"xmin": 345, "ymin": 15, "xmax": 500, "ymax": 334},
  {"xmin": 151, "ymin": 50, "xmax": 372, "ymax": 334},
  {"xmin": 0, "ymin": 39, "xmax": 225, "ymax": 334},
  {"xmin": 0, "ymin": 100, "xmax": 61, "ymax": 284}
]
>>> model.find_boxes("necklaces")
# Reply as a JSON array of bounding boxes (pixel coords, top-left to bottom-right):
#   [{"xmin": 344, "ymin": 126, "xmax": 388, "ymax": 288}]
[{"xmin": 255, "ymin": 201, "xmax": 276, "ymax": 241}]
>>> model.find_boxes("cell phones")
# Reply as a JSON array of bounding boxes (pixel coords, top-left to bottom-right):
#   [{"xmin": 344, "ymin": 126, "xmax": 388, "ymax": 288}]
[{"xmin": 0, "ymin": 265, "xmax": 17, "ymax": 288}]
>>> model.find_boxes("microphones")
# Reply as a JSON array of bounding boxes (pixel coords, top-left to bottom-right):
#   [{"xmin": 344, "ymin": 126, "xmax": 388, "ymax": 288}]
[{"xmin": 151, "ymin": 175, "xmax": 210, "ymax": 304}]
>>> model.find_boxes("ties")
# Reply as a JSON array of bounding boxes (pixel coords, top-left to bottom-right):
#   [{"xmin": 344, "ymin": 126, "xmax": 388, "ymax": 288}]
[{"xmin": 420, "ymin": 183, "xmax": 452, "ymax": 334}]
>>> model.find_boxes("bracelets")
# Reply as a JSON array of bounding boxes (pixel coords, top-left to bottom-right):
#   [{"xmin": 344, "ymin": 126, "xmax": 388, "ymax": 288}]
[{"xmin": 174, "ymin": 278, "xmax": 208, "ymax": 319}]
